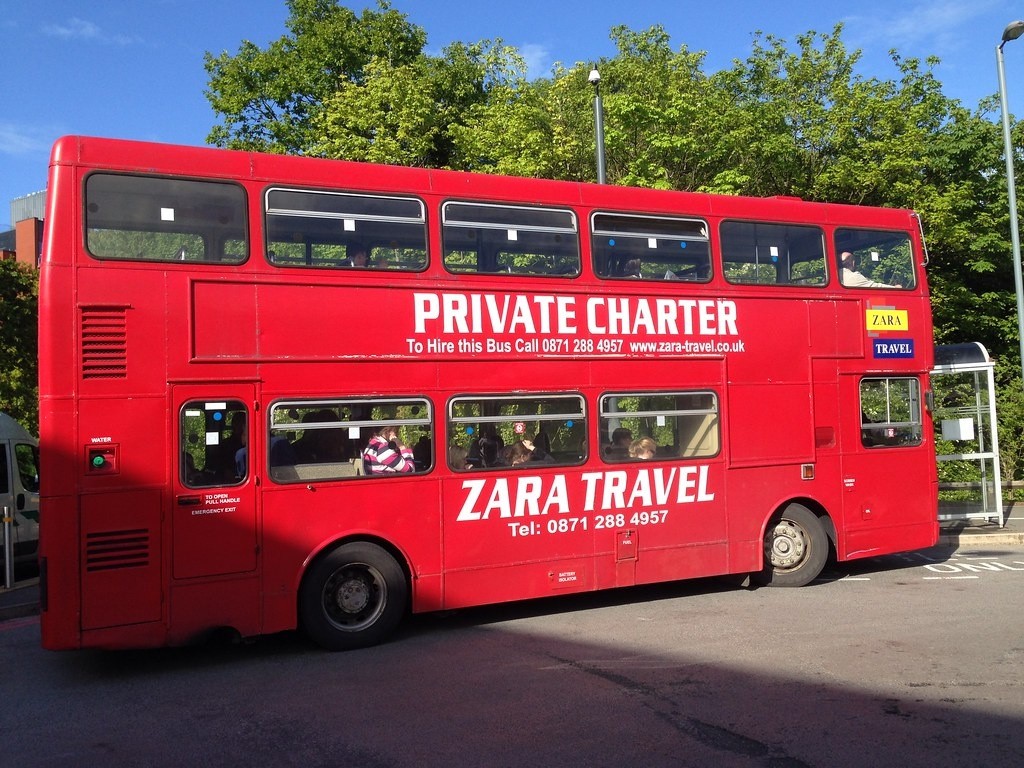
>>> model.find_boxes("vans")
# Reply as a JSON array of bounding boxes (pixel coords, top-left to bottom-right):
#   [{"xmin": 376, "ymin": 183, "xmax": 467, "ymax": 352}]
[{"xmin": 0, "ymin": 412, "xmax": 39, "ymax": 564}]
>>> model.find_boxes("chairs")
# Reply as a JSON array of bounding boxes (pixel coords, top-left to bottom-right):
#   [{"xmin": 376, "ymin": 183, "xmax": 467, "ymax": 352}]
[{"xmin": 349, "ymin": 447, "xmax": 367, "ymax": 476}]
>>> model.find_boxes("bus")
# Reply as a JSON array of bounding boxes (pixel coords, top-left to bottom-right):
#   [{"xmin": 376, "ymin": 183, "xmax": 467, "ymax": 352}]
[{"xmin": 37, "ymin": 137, "xmax": 940, "ymax": 657}]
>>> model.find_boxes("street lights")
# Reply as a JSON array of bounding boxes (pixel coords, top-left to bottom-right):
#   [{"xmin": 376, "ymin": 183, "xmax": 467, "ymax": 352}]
[
  {"xmin": 996, "ymin": 18, "xmax": 1021, "ymax": 360},
  {"xmin": 587, "ymin": 68, "xmax": 606, "ymax": 184}
]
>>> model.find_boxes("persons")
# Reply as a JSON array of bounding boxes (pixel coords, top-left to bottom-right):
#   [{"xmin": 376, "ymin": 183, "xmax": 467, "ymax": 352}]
[
  {"xmin": 862, "ymin": 408, "xmax": 873, "ymax": 438},
  {"xmin": 183, "ymin": 409, "xmax": 657, "ymax": 487},
  {"xmin": 338, "ymin": 241, "xmax": 390, "ymax": 269},
  {"xmin": 616, "ymin": 253, "xmax": 643, "ymax": 280},
  {"xmin": 839, "ymin": 250, "xmax": 902, "ymax": 289}
]
